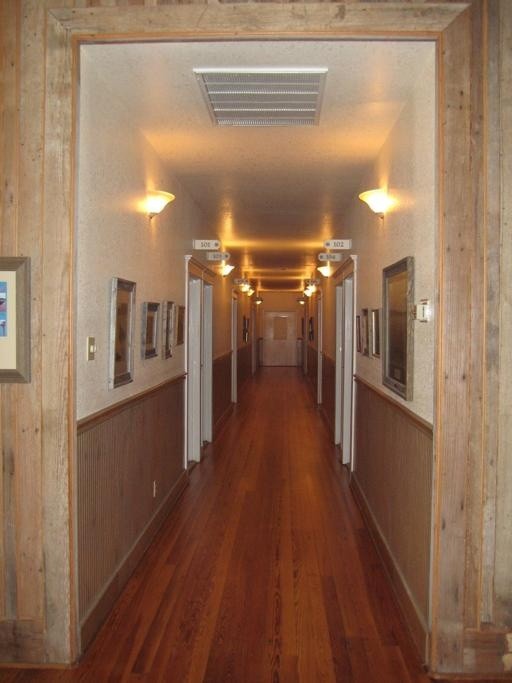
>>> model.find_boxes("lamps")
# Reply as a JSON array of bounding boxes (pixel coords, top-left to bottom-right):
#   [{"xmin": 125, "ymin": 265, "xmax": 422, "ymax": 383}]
[
  {"xmin": 223, "ymin": 264, "xmax": 236, "ymax": 277},
  {"xmin": 145, "ymin": 188, "xmax": 175, "ymax": 218},
  {"xmin": 317, "ymin": 265, "xmax": 329, "ymax": 277},
  {"xmin": 358, "ymin": 189, "xmax": 388, "ymax": 219},
  {"xmin": 233, "ymin": 278, "xmax": 263, "ymax": 304},
  {"xmin": 296, "ymin": 277, "xmax": 320, "ymax": 305}
]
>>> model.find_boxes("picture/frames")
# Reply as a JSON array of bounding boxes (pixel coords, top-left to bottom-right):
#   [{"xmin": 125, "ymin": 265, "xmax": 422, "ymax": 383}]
[
  {"xmin": 163, "ymin": 301, "xmax": 174, "ymax": 360},
  {"xmin": 0, "ymin": 256, "xmax": 31, "ymax": 383},
  {"xmin": 142, "ymin": 301, "xmax": 159, "ymax": 360},
  {"xmin": 382, "ymin": 256, "xmax": 416, "ymax": 401},
  {"xmin": 356, "ymin": 307, "xmax": 382, "ymax": 360},
  {"xmin": 109, "ymin": 276, "xmax": 137, "ymax": 390},
  {"xmin": 176, "ymin": 304, "xmax": 184, "ymax": 347}
]
